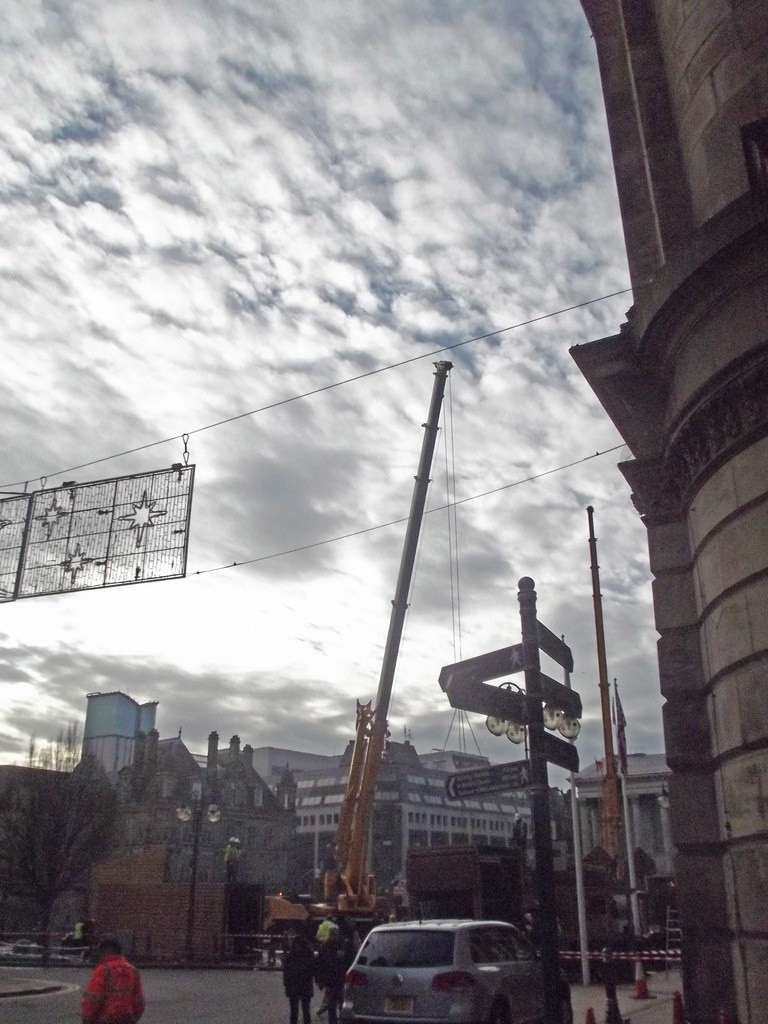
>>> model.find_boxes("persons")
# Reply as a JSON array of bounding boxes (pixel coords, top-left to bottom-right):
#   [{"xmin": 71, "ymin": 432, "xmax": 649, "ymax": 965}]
[
  {"xmin": 263, "ymin": 923, "xmax": 277, "ymax": 969},
  {"xmin": 514, "ymin": 813, "xmax": 527, "ymax": 868},
  {"xmin": 81, "ymin": 939, "xmax": 145, "ymax": 1024},
  {"xmin": 282, "ymin": 911, "xmax": 363, "ymax": 1024},
  {"xmin": 224, "ymin": 837, "xmax": 242, "ymax": 884}
]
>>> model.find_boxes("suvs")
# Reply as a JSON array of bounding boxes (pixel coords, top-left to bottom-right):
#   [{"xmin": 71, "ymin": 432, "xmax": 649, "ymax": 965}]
[{"xmin": 338, "ymin": 919, "xmax": 576, "ymax": 1024}]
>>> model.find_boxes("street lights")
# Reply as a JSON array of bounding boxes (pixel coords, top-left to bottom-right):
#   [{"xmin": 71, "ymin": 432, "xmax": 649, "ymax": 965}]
[{"xmin": 175, "ymin": 791, "xmax": 222, "ymax": 970}]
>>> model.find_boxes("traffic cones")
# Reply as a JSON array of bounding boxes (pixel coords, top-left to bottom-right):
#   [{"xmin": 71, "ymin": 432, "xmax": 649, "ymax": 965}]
[
  {"xmin": 628, "ymin": 961, "xmax": 658, "ymax": 1000},
  {"xmin": 671, "ymin": 990, "xmax": 691, "ymax": 1024},
  {"xmin": 585, "ymin": 1007, "xmax": 597, "ymax": 1024}
]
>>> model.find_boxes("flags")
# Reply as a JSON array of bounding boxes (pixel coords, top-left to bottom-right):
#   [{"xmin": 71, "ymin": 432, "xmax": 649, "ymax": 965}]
[{"xmin": 614, "ymin": 682, "xmax": 630, "ymax": 777}]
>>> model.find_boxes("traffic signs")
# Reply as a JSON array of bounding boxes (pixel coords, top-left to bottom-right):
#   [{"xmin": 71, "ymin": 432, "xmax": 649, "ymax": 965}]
[
  {"xmin": 545, "ymin": 730, "xmax": 580, "ymax": 774},
  {"xmin": 540, "ymin": 672, "xmax": 583, "ymax": 719},
  {"xmin": 443, "ymin": 759, "xmax": 532, "ymax": 801},
  {"xmin": 537, "ymin": 618, "xmax": 574, "ymax": 673},
  {"xmin": 444, "ymin": 674, "xmax": 529, "ymax": 727},
  {"xmin": 437, "ymin": 642, "xmax": 524, "ymax": 693}
]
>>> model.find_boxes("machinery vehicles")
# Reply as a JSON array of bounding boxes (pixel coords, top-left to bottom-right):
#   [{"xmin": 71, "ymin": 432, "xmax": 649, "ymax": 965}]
[{"xmin": 255, "ymin": 358, "xmax": 457, "ymax": 975}]
